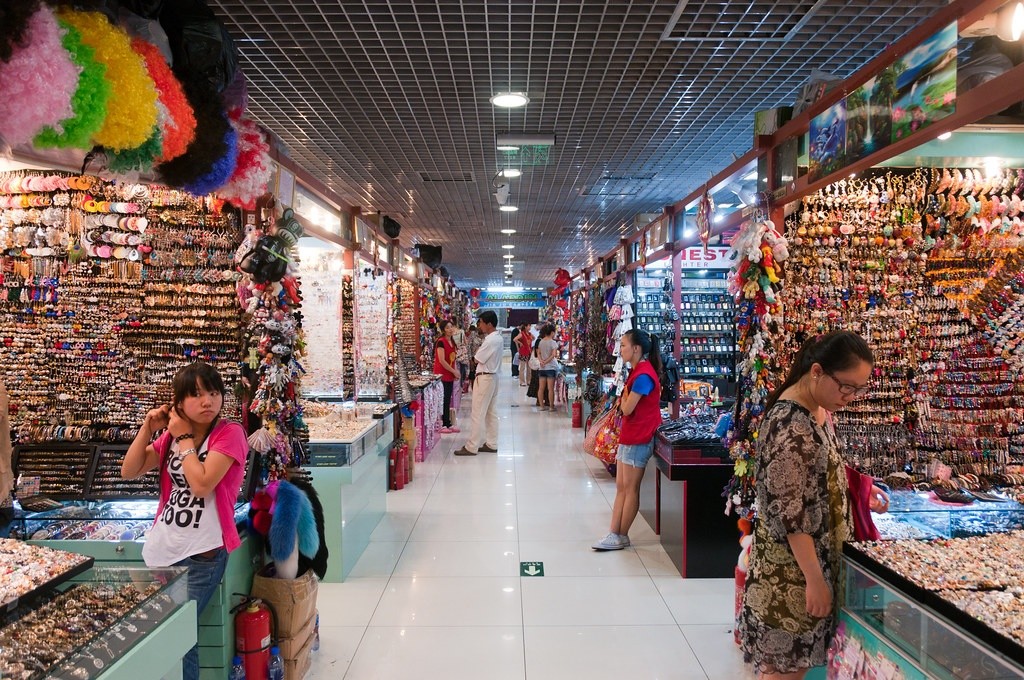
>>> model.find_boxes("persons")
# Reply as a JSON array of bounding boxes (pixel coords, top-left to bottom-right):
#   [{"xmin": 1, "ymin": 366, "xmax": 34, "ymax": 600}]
[
  {"xmin": 468, "ymin": 320, "xmax": 485, "ymax": 389},
  {"xmin": 119, "ymin": 363, "xmax": 249, "ymax": 680},
  {"xmin": 592, "ymin": 328, "xmax": 661, "ymax": 549},
  {"xmin": 739, "ymin": 331, "xmax": 890, "ymax": 679},
  {"xmin": 433, "ymin": 320, "xmax": 461, "ymax": 433},
  {"xmin": 511, "ymin": 321, "xmax": 559, "ymax": 412},
  {"xmin": 454, "ymin": 311, "xmax": 503, "ymax": 456}
]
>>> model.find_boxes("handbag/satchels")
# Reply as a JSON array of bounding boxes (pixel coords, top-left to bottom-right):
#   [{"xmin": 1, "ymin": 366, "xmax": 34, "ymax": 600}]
[
  {"xmin": 528, "ymin": 349, "xmax": 540, "ymax": 370},
  {"xmin": 844, "ymin": 463, "xmax": 881, "ymax": 541},
  {"xmin": 583, "ymin": 404, "xmax": 622, "ymax": 464}
]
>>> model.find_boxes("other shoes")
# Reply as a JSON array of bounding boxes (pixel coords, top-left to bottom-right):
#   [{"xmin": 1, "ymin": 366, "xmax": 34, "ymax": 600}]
[
  {"xmin": 478, "ymin": 443, "xmax": 497, "ymax": 452},
  {"xmin": 441, "ymin": 426, "xmax": 452, "ymax": 433},
  {"xmin": 540, "ymin": 407, "xmax": 544, "ymax": 411},
  {"xmin": 549, "ymin": 407, "xmax": 557, "ymax": 412},
  {"xmin": 450, "ymin": 425, "xmax": 460, "ymax": 433},
  {"xmin": 453, "ymin": 446, "xmax": 476, "ymax": 456}
]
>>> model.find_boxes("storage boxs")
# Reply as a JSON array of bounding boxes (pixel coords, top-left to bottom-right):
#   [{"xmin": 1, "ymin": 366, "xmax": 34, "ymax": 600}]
[
  {"xmin": 250, "ymin": 559, "xmax": 319, "ymax": 680},
  {"xmin": 400, "ymin": 417, "xmax": 418, "ymax": 481}
]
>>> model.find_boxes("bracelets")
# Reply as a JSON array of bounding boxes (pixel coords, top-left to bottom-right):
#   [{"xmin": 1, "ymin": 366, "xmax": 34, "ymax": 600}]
[
  {"xmin": 28, "ymin": 425, "xmax": 139, "ymax": 442},
  {"xmin": 176, "ymin": 433, "xmax": 193, "ymax": 444},
  {"xmin": 37, "ymin": 507, "xmax": 154, "ymax": 540}
]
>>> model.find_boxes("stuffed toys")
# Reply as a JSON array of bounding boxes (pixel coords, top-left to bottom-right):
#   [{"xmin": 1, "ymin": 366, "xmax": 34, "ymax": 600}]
[
  {"xmin": 253, "ymin": 480, "xmax": 319, "ymax": 579},
  {"xmin": 717, "ymin": 213, "xmax": 788, "ymax": 580}
]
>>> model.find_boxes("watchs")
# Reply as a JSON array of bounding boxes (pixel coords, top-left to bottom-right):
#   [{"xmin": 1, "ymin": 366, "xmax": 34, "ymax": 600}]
[{"xmin": 178, "ymin": 448, "xmax": 197, "ymax": 460}]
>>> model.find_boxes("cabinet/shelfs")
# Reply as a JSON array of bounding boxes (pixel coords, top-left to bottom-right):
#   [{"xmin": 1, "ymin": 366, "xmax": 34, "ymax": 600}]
[{"xmin": 635, "ymin": 266, "xmax": 738, "ymax": 406}]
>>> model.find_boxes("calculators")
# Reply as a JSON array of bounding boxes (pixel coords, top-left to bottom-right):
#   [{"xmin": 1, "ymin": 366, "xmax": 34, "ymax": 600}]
[
  {"xmin": 934, "ymin": 489, "xmax": 976, "ymax": 504},
  {"xmin": 18, "ymin": 494, "xmax": 63, "ymax": 512},
  {"xmin": 957, "ymin": 486, "xmax": 1007, "ymax": 502}
]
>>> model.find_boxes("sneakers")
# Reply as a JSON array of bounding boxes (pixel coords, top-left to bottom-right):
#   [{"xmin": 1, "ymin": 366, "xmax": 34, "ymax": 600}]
[
  {"xmin": 619, "ymin": 534, "xmax": 631, "ymax": 547},
  {"xmin": 591, "ymin": 531, "xmax": 624, "ymax": 549}
]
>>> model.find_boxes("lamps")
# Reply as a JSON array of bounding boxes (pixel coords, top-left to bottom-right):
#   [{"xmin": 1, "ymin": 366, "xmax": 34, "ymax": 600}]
[
  {"xmin": 958, "ymin": 0, "xmax": 1024, "ymax": 42},
  {"xmin": 728, "ymin": 182, "xmax": 753, "ymax": 205}
]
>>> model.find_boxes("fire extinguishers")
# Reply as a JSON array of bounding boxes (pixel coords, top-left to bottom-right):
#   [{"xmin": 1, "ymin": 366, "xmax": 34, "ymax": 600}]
[
  {"xmin": 584, "ymin": 415, "xmax": 593, "ymax": 439},
  {"xmin": 229, "ymin": 593, "xmax": 279, "ymax": 680},
  {"xmin": 735, "ymin": 565, "xmax": 750, "ymax": 646},
  {"xmin": 389, "ymin": 439, "xmax": 404, "ymax": 491},
  {"xmin": 572, "ymin": 395, "xmax": 582, "ymax": 428},
  {"xmin": 398, "ymin": 438, "xmax": 409, "ymax": 484}
]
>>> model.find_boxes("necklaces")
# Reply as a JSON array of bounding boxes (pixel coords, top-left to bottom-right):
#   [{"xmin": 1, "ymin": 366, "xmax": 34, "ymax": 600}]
[{"xmin": 0, "ymin": 583, "xmax": 170, "ymax": 679}]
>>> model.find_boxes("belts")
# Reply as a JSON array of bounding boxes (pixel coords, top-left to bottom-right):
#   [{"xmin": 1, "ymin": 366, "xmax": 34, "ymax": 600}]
[{"xmin": 197, "ymin": 550, "xmax": 215, "ymax": 559}]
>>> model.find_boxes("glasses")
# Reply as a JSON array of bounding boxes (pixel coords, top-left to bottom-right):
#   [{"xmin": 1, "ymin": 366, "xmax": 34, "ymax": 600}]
[{"xmin": 825, "ymin": 370, "xmax": 870, "ymax": 396}]
